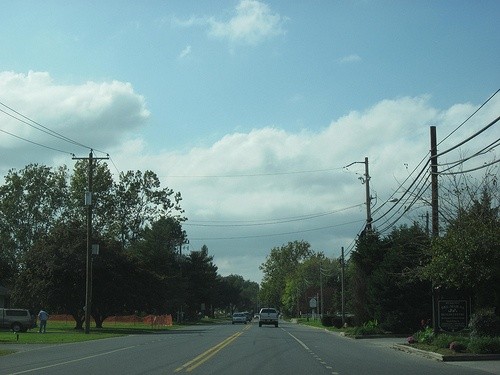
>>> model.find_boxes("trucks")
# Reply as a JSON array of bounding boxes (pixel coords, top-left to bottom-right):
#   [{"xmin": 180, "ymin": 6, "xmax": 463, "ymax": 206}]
[{"xmin": 0, "ymin": 308, "xmax": 37, "ymax": 332}]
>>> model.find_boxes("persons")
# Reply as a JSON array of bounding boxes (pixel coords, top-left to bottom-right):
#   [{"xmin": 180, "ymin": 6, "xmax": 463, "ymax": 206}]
[{"xmin": 38, "ymin": 309, "xmax": 49, "ymax": 333}]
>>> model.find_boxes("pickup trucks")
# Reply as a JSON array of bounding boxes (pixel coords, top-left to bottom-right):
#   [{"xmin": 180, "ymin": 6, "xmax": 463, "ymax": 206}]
[
  {"xmin": 232, "ymin": 313, "xmax": 247, "ymax": 325},
  {"xmin": 258, "ymin": 308, "xmax": 278, "ymax": 327}
]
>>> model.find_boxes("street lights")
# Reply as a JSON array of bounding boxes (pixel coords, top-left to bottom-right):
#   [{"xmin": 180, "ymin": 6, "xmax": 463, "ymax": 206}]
[{"xmin": 367, "ymin": 198, "xmax": 399, "ymax": 231}]
